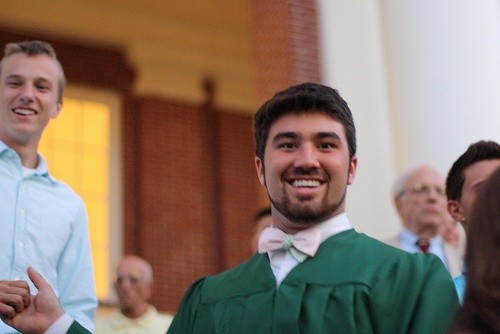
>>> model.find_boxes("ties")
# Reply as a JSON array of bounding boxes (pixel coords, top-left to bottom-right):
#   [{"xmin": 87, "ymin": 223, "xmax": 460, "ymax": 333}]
[{"xmin": 419, "ymin": 240, "xmax": 430, "ymax": 254}]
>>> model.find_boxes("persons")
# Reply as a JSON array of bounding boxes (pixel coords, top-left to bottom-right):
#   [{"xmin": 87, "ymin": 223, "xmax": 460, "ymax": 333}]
[
  {"xmin": 2, "ymin": 81, "xmax": 458, "ymax": 334},
  {"xmin": 254, "ymin": 206, "xmax": 273, "ymax": 253},
  {"xmin": 0, "ymin": 39, "xmax": 98, "ymax": 334},
  {"xmin": 92, "ymin": 253, "xmax": 176, "ymax": 333},
  {"xmin": 387, "ymin": 139, "xmax": 500, "ymax": 334}
]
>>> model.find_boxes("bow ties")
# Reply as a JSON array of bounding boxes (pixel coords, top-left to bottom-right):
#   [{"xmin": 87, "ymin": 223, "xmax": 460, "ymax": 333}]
[{"xmin": 257, "ymin": 227, "xmax": 322, "ymax": 263}]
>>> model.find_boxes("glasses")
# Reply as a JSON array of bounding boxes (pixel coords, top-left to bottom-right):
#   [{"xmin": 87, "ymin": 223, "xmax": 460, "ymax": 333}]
[{"xmin": 400, "ymin": 183, "xmax": 446, "ymax": 197}]
